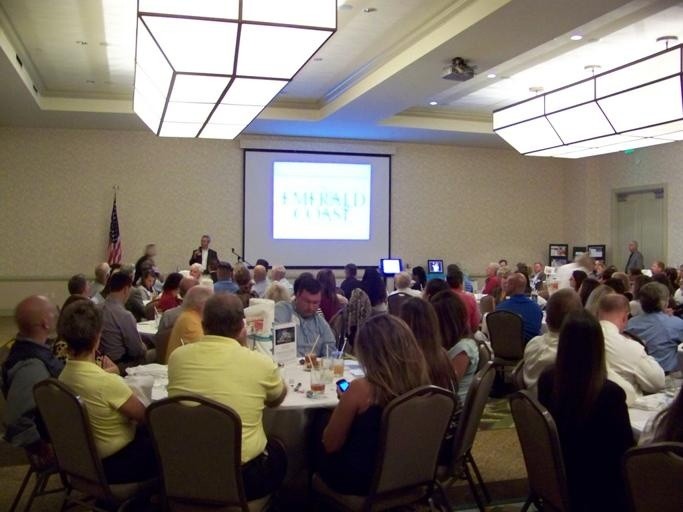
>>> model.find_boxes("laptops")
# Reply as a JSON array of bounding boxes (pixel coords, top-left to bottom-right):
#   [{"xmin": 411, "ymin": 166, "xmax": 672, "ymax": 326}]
[{"xmin": 380, "ymin": 259, "xmax": 403, "ymax": 275}]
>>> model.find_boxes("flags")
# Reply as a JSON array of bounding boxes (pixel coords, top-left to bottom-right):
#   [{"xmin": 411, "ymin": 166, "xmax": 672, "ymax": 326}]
[{"xmin": 109, "ymin": 193, "xmax": 122, "ymax": 264}]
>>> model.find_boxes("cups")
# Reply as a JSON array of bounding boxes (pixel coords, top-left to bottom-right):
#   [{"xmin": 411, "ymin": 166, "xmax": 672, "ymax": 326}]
[
  {"xmin": 304, "ymin": 353, "xmax": 345, "ymax": 396},
  {"xmin": 155, "ymin": 314, "xmax": 162, "ymax": 328}
]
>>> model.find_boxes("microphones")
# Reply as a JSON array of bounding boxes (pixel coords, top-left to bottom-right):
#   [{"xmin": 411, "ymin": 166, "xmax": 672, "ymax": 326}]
[{"xmin": 231, "ymin": 247, "xmax": 253, "ymax": 268}]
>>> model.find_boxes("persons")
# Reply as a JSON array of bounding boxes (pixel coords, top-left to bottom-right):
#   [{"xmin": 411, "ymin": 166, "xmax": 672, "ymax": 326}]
[
  {"xmin": 481, "ymin": 239, "xmax": 683, "ymax": 512},
  {"xmin": 135, "ymin": 245, "xmax": 157, "ymax": 276},
  {"xmin": 58, "ymin": 299, "xmax": 161, "ymax": 486},
  {"xmin": 0, "ymin": 295, "xmax": 66, "ymax": 463},
  {"xmin": 159, "ymin": 232, "xmax": 482, "ymax": 511},
  {"xmin": 62, "ymin": 261, "xmax": 160, "ymax": 369}
]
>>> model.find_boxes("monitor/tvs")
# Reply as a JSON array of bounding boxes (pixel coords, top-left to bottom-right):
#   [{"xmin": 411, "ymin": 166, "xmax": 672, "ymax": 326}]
[{"xmin": 427, "ymin": 259, "xmax": 444, "ymax": 273}]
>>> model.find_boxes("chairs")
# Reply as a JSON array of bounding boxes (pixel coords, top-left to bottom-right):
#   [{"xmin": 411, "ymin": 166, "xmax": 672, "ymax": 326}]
[
  {"xmin": 479, "ymin": 295, "xmax": 495, "ymax": 316},
  {"xmin": 33, "ymin": 379, "xmax": 164, "ymax": 512},
  {"xmin": 623, "ymin": 330, "xmax": 647, "ymax": 353},
  {"xmin": 146, "ymin": 393, "xmax": 272, "ymax": 511},
  {"xmin": 509, "ymin": 390, "xmax": 574, "ymax": 512},
  {"xmin": 312, "ymin": 385, "xmax": 458, "ymax": 512},
  {"xmin": 485, "ymin": 310, "xmax": 524, "ymax": 388},
  {"xmin": 435, "ymin": 360, "xmax": 496, "ymax": 511},
  {"xmin": 622, "ymin": 442, "xmax": 683, "ymax": 511},
  {"xmin": 7, "ymin": 448, "xmax": 67, "ymax": 511},
  {"xmin": 510, "ymin": 359, "xmax": 524, "ymax": 389}
]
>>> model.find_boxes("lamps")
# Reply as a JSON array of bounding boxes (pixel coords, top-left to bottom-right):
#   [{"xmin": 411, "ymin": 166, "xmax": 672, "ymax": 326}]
[
  {"xmin": 490, "ymin": 35, "xmax": 683, "ymax": 161},
  {"xmin": 132, "ymin": 0, "xmax": 337, "ymax": 141}
]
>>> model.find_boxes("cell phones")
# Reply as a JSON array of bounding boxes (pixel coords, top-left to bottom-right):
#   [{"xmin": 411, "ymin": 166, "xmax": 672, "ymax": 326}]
[{"xmin": 336, "ymin": 379, "xmax": 351, "ymax": 392}]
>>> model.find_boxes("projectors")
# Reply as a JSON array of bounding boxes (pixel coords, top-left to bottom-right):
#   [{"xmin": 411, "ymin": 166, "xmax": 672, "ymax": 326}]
[{"xmin": 442, "ymin": 57, "xmax": 474, "ymax": 82}]
